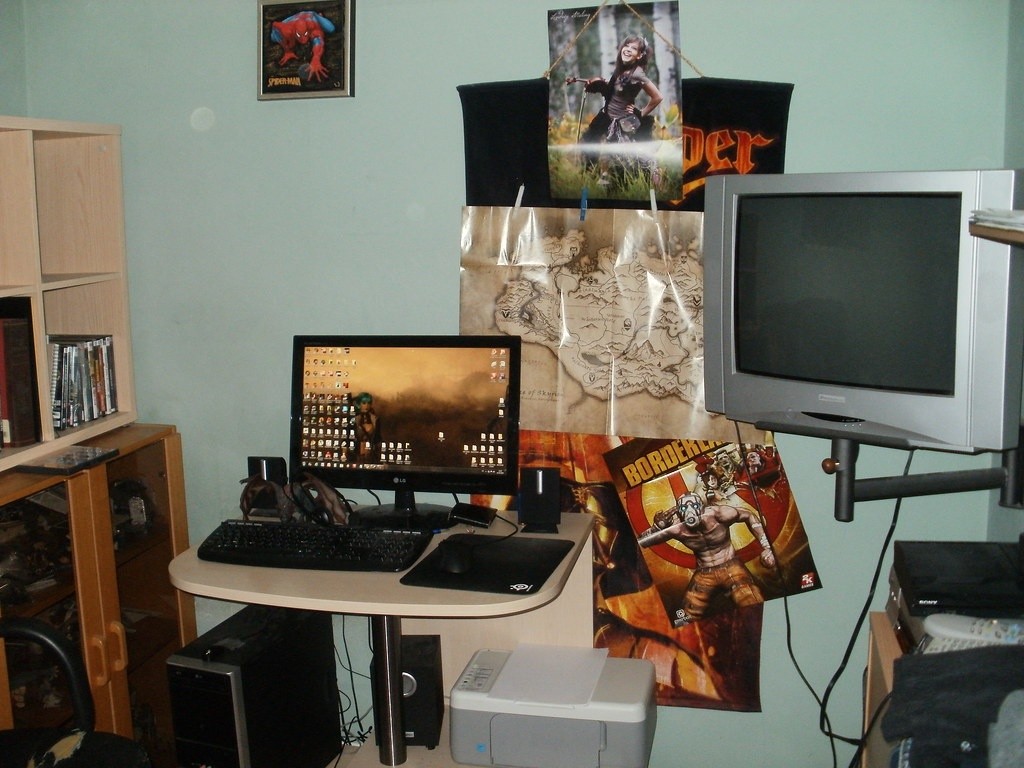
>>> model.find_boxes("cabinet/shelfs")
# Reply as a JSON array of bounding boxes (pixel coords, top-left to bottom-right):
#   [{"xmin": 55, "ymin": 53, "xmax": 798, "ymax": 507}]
[
  {"xmin": 1, "ymin": 424, "xmax": 203, "ymax": 768},
  {"xmin": 1, "ymin": 107, "xmax": 141, "ymax": 476}
]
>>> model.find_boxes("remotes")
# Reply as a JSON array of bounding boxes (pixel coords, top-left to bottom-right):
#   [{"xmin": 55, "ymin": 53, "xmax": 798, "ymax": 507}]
[{"xmin": 923, "ymin": 613, "xmax": 1024, "ymax": 646}]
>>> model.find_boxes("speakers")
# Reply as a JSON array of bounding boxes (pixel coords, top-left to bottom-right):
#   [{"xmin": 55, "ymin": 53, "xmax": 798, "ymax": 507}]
[
  {"xmin": 247, "ymin": 457, "xmax": 287, "ymax": 487},
  {"xmin": 370, "ymin": 634, "xmax": 445, "ymax": 751},
  {"xmin": 518, "ymin": 467, "xmax": 562, "ymax": 534}
]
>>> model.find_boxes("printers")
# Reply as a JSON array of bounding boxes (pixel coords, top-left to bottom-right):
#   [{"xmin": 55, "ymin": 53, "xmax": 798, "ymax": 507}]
[{"xmin": 448, "ymin": 648, "xmax": 659, "ymax": 768}]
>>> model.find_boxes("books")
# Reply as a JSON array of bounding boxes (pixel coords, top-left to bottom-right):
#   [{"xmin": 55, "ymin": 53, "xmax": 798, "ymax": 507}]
[
  {"xmin": 45, "ymin": 334, "xmax": 120, "ymax": 433},
  {"xmin": 0, "ymin": 318, "xmax": 38, "ymax": 448}
]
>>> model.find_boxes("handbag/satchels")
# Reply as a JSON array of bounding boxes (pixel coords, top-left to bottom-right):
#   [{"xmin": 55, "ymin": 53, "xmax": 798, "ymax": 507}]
[{"xmin": 617, "ymin": 114, "xmax": 641, "ymax": 136}]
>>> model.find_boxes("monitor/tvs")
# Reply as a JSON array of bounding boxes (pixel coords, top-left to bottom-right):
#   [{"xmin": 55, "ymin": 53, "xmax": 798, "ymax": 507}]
[
  {"xmin": 703, "ymin": 170, "xmax": 1024, "ymax": 455},
  {"xmin": 288, "ymin": 334, "xmax": 522, "ymax": 495}
]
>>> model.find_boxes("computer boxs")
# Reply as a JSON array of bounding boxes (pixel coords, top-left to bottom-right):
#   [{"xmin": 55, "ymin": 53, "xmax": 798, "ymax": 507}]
[{"xmin": 165, "ymin": 602, "xmax": 343, "ymax": 768}]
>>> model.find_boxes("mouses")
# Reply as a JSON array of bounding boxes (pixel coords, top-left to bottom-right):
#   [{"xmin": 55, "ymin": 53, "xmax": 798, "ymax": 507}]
[{"xmin": 439, "ymin": 539, "xmax": 474, "ymax": 576}]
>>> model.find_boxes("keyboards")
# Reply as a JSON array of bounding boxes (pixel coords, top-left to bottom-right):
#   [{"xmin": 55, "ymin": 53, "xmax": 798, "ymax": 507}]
[{"xmin": 196, "ymin": 519, "xmax": 435, "ymax": 573}]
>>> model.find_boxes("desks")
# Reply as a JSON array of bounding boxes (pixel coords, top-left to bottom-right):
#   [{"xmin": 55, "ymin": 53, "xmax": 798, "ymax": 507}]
[
  {"xmin": 166, "ymin": 508, "xmax": 598, "ymax": 768},
  {"xmin": 856, "ymin": 610, "xmax": 906, "ymax": 768}
]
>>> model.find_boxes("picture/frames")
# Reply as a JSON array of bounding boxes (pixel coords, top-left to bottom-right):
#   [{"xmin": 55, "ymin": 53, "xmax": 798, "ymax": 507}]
[{"xmin": 256, "ymin": 0, "xmax": 357, "ymax": 102}]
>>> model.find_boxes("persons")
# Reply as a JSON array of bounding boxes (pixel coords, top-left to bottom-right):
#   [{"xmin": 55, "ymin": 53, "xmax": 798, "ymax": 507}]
[{"xmin": 576, "ymin": 34, "xmax": 670, "ymax": 197}]
